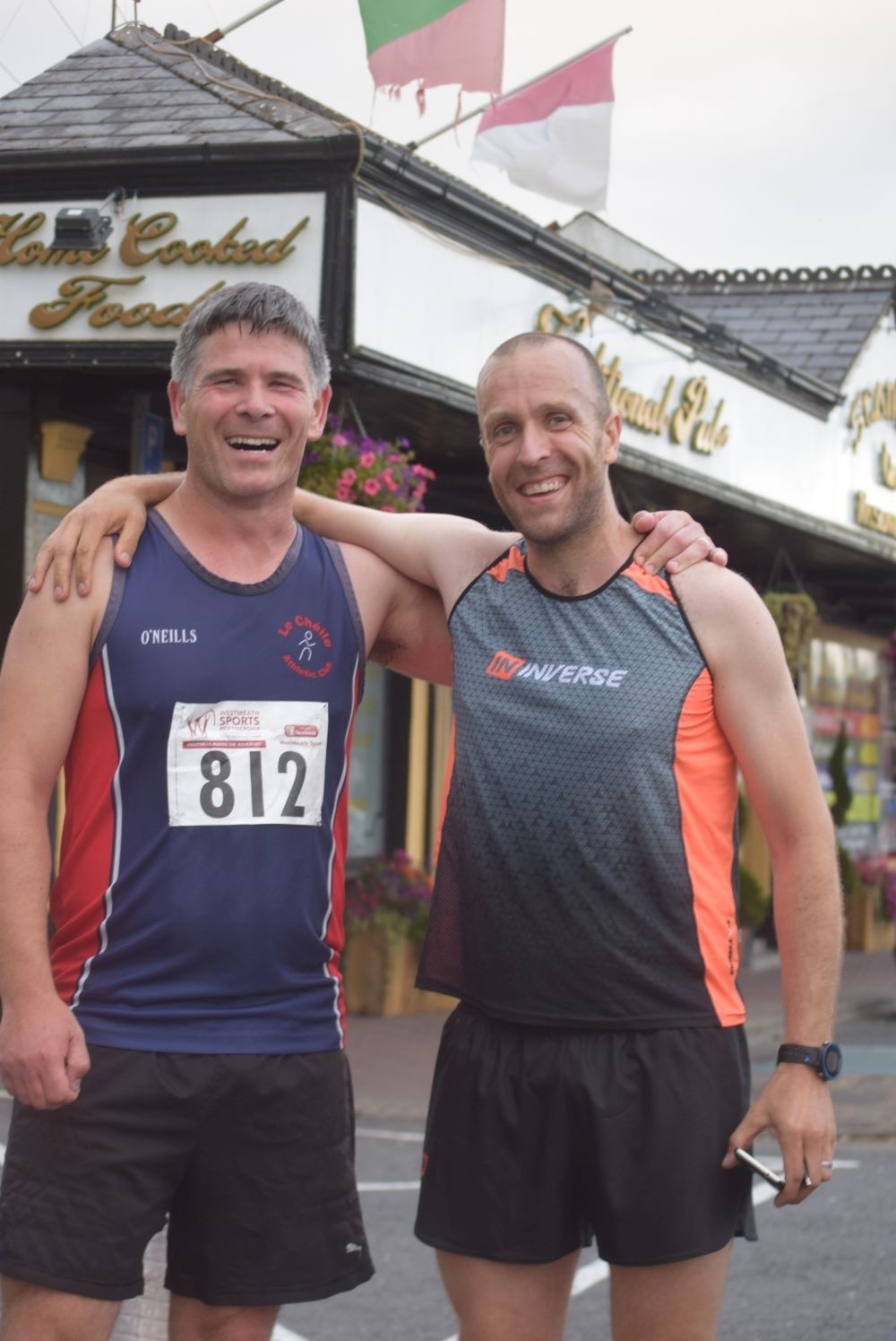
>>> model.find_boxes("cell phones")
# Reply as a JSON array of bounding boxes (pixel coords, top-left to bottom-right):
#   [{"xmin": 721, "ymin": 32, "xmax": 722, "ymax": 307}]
[{"xmin": 734, "ymin": 1146, "xmax": 789, "ymax": 1190}]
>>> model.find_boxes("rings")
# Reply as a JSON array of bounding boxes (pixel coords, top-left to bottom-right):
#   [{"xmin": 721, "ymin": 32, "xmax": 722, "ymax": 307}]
[{"xmin": 821, "ymin": 1161, "xmax": 834, "ymax": 1169}]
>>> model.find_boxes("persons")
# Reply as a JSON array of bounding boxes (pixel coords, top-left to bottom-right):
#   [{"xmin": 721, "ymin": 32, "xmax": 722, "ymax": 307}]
[
  {"xmin": 1, "ymin": 278, "xmax": 733, "ymax": 1341},
  {"xmin": 26, "ymin": 332, "xmax": 843, "ymax": 1340}
]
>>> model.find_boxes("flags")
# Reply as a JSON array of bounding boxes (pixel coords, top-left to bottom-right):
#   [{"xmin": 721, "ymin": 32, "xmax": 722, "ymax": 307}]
[
  {"xmin": 473, "ymin": 35, "xmax": 620, "ymax": 215},
  {"xmin": 356, "ymin": 0, "xmax": 506, "ymax": 141}
]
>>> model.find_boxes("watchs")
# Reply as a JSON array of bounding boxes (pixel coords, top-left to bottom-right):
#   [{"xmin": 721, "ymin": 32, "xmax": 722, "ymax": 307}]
[{"xmin": 776, "ymin": 1041, "xmax": 841, "ymax": 1082}]
